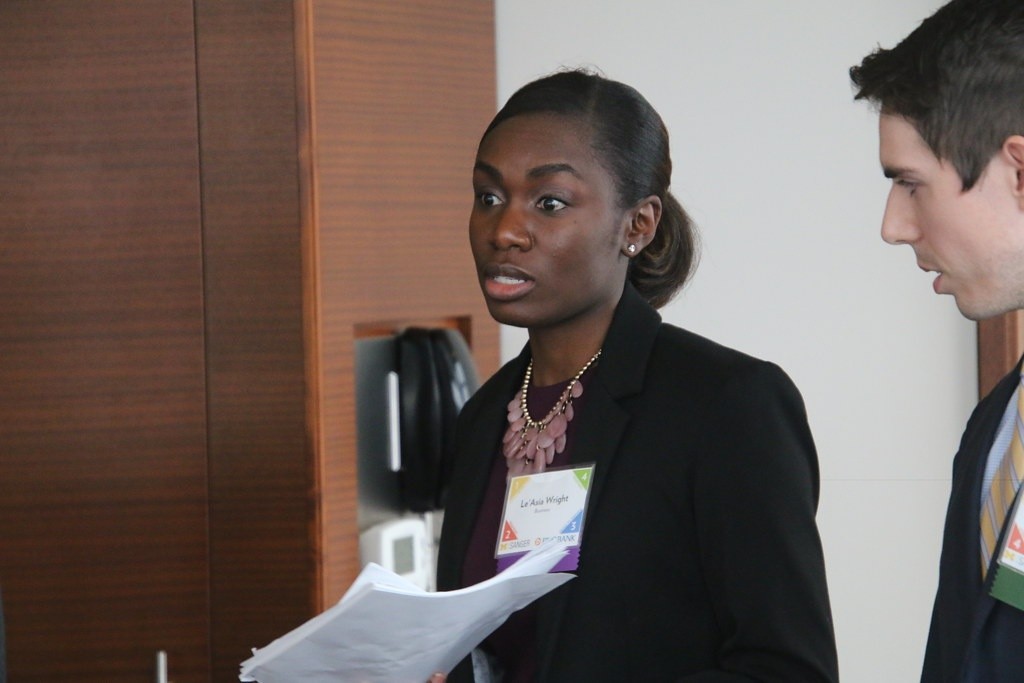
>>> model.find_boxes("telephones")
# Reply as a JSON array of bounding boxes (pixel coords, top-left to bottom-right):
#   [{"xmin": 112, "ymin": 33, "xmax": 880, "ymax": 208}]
[{"xmin": 392, "ymin": 324, "xmax": 484, "ymax": 514}]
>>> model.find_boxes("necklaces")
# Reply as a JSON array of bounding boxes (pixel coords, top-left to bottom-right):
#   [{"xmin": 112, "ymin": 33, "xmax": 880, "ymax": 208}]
[{"xmin": 503, "ymin": 348, "xmax": 602, "ymax": 489}]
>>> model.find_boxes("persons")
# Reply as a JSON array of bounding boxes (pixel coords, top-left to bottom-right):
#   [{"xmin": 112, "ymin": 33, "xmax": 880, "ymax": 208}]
[
  {"xmin": 849, "ymin": 0, "xmax": 1024, "ymax": 682},
  {"xmin": 436, "ymin": 70, "xmax": 840, "ymax": 683}
]
